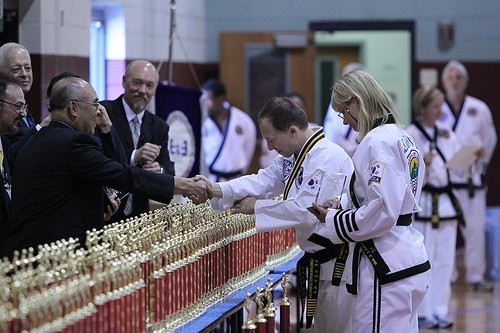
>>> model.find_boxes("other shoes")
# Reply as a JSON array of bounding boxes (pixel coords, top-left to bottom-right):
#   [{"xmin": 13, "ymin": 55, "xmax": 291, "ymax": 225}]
[
  {"xmin": 437, "ymin": 316, "xmax": 453, "ymax": 327},
  {"xmin": 472, "ymin": 281, "xmax": 492, "ymax": 290},
  {"xmin": 418, "ymin": 315, "xmax": 438, "ymax": 329}
]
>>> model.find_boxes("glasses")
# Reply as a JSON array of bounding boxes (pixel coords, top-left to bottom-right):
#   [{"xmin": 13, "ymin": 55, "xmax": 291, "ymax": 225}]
[
  {"xmin": 338, "ymin": 99, "xmax": 350, "ymax": 120},
  {"xmin": 71, "ymin": 99, "xmax": 99, "ymax": 111},
  {"xmin": 0, "ymin": 99, "xmax": 29, "ymax": 111}
]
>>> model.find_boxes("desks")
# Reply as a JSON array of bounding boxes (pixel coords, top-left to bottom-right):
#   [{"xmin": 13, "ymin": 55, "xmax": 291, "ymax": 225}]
[{"xmin": 174, "ymin": 251, "xmax": 304, "ymax": 333}]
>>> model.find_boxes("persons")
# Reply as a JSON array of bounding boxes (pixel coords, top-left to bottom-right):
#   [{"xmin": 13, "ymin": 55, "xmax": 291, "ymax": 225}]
[
  {"xmin": 0, "ymin": 194, "xmax": 291, "ymax": 333},
  {"xmin": 0, "ymin": 43, "xmax": 366, "ymax": 273},
  {"xmin": 312, "ymin": 71, "xmax": 431, "ymax": 333},
  {"xmin": 438, "ymin": 59, "xmax": 497, "ymax": 290},
  {"xmin": 182, "ymin": 97, "xmax": 356, "ymax": 333},
  {"xmin": 404, "ymin": 85, "xmax": 485, "ymax": 328}
]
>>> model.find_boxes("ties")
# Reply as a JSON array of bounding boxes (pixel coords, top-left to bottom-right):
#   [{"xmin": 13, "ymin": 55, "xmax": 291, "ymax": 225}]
[{"xmin": 123, "ymin": 116, "xmax": 140, "ymax": 216}]
